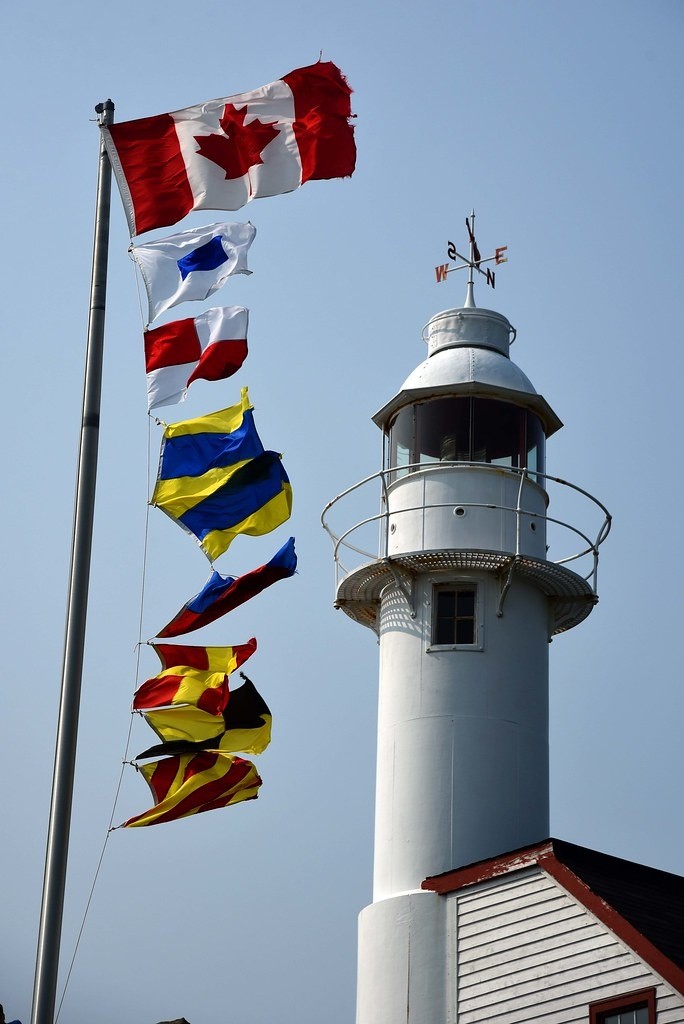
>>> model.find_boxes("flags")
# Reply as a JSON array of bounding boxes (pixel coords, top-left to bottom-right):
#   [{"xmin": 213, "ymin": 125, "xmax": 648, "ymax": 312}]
[
  {"xmin": 143, "ymin": 306, "xmax": 249, "ymax": 411},
  {"xmin": 118, "ymin": 637, "xmax": 272, "ymax": 828},
  {"xmin": 99, "ymin": 50, "xmax": 358, "ymax": 238},
  {"xmin": 156, "ymin": 537, "xmax": 299, "ymax": 638},
  {"xmin": 131, "ymin": 221, "xmax": 257, "ymax": 327},
  {"xmin": 150, "ymin": 388, "xmax": 293, "ymax": 563}
]
